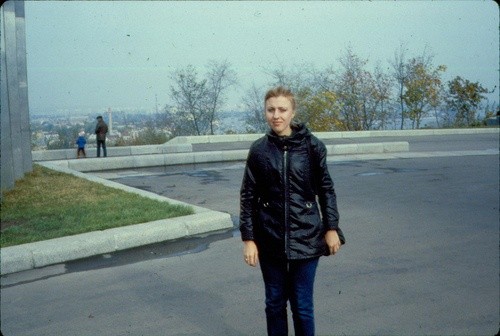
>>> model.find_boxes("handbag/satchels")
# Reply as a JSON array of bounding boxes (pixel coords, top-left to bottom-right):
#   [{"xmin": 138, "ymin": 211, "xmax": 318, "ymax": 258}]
[{"xmin": 323, "ymin": 219, "xmax": 345, "ymax": 257}]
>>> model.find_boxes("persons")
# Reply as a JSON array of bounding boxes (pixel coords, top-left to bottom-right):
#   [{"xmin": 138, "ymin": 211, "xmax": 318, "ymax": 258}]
[
  {"xmin": 76, "ymin": 131, "xmax": 88, "ymax": 159},
  {"xmin": 94, "ymin": 115, "xmax": 108, "ymax": 157},
  {"xmin": 239, "ymin": 87, "xmax": 342, "ymax": 336}
]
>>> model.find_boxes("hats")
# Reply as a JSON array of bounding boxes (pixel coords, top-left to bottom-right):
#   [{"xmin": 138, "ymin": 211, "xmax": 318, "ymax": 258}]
[
  {"xmin": 96, "ymin": 116, "xmax": 102, "ymax": 119},
  {"xmin": 78, "ymin": 131, "xmax": 85, "ymax": 137}
]
